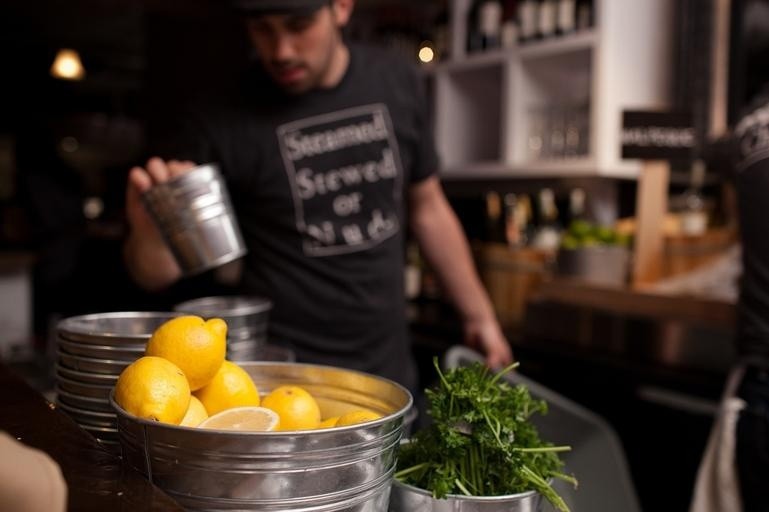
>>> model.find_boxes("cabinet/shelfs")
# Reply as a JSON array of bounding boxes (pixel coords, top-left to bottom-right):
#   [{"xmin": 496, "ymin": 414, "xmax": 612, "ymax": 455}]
[{"xmin": 432, "ymin": 0, "xmax": 734, "ymax": 364}]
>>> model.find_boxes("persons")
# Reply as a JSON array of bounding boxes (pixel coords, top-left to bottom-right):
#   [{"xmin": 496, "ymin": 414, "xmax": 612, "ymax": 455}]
[
  {"xmin": 0, "ymin": 360, "xmax": 155, "ymax": 512},
  {"xmin": 120, "ymin": 0, "xmax": 517, "ymax": 407}
]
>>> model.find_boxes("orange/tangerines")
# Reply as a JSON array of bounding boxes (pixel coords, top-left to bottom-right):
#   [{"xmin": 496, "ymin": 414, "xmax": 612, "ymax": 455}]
[{"xmin": 558, "ymin": 218, "xmax": 631, "ymax": 251}]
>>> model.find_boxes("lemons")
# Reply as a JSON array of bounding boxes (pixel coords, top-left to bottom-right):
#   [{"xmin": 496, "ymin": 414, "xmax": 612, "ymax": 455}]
[{"xmin": 114, "ymin": 315, "xmax": 381, "ymax": 433}]
